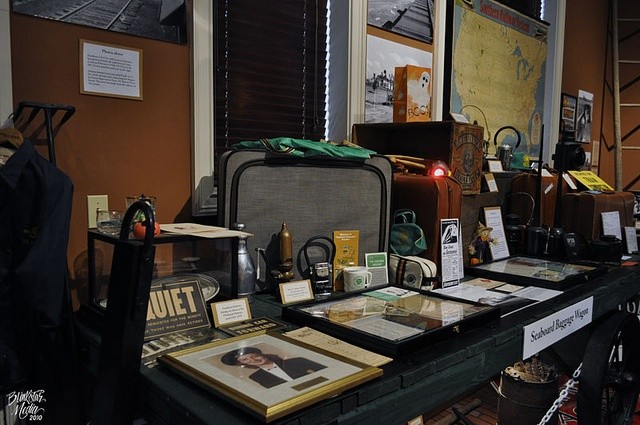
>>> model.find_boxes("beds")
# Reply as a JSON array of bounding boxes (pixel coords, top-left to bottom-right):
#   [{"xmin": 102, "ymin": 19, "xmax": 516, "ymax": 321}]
[{"xmin": 77, "ymin": 222, "xmax": 243, "ymax": 349}]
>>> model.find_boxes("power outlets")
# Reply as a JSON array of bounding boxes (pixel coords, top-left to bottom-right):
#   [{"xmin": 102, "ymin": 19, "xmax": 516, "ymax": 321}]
[{"xmin": 86, "ymin": 194, "xmax": 111, "ymax": 229}]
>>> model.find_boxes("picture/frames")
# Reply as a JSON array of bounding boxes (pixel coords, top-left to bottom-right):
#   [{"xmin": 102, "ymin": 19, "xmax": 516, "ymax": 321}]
[
  {"xmin": 561, "ymin": 107, "xmax": 576, "ymax": 119},
  {"xmin": 576, "ymin": 97, "xmax": 593, "ymax": 144},
  {"xmin": 157, "ymin": 328, "xmax": 383, "ymax": 423},
  {"xmin": 560, "ymin": 120, "xmax": 575, "ymax": 133},
  {"xmin": 562, "ymin": 93, "xmax": 577, "ymax": 108}
]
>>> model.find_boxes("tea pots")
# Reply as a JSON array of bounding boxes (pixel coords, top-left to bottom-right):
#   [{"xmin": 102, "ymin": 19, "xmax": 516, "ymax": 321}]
[{"xmin": 495, "ymin": 126, "xmax": 522, "ymax": 170}]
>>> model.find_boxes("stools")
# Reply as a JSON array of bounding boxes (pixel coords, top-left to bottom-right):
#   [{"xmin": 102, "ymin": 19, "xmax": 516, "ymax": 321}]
[{"xmin": 504, "ymin": 213, "xmax": 520, "ymax": 253}]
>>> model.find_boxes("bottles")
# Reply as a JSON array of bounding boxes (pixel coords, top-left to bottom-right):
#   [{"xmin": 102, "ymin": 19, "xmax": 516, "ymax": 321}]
[{"xmin": 497, "ymin": 144, "xmax": 511, "ymax": 171}]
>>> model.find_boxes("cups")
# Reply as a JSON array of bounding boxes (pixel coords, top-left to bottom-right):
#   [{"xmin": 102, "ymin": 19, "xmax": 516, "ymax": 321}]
[
  {"xmin": 97, "ymin": 210, "xmax": 125, "ymax": 235},
  {"xmin": 128, "ymin": 197, "xmax": 154, "ymax": 226},
  {"xmin": 341, "ymin": 265, "xmax": 371, "ymax": 292}
]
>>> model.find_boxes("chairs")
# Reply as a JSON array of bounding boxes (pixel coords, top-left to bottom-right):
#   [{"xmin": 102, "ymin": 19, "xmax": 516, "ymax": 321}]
[{"xmin": 500, "ymin": 192, "xmax": 535, "ymax": 256}]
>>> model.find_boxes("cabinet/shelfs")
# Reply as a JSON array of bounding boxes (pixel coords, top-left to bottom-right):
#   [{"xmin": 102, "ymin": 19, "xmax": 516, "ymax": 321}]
[{"xmin": 512, "ymin": 172, "xmax": 560, "ymax": 227}]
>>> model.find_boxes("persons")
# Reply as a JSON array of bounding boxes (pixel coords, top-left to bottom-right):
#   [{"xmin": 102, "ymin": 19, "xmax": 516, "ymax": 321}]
[
  {"xmin": 223, "ymin": 345, "xmax": 328, "ymax": 391},
  {"xmin": 576, "ymin": 103, "xmax": 592, "ymax": 144}
]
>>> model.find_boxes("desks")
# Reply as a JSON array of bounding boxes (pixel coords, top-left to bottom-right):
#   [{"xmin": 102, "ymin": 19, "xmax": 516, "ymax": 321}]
[{"xmin": 142, "ymin": 250, "xmax": 639, "ymax": 425}]
